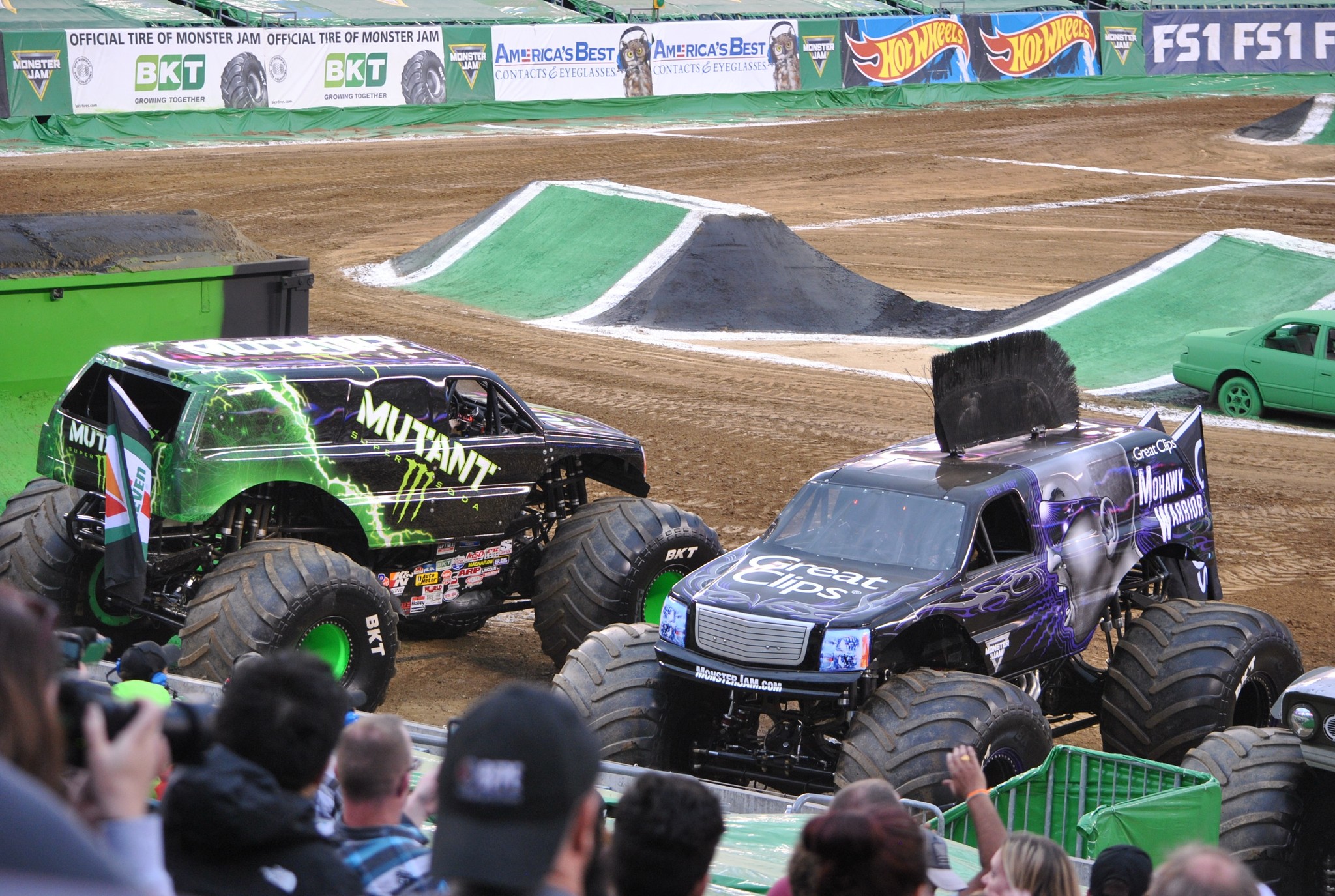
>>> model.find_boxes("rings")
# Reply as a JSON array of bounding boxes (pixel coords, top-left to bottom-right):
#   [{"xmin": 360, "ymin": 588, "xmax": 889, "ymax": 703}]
[{"xmin": 961, "ymin": 754, "xmax": 971, "ymax": 761}]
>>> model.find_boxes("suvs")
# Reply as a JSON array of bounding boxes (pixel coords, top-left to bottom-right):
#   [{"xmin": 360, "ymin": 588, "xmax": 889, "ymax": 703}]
[
  {"xmin": 1184, "ymin": 664, "xmax": 1335, "ymax": 896},
  {"xmin": 553, "ymin": 404, "xmax": 1306, "ymax": 816},
  {"xmin": 0, "ymin": 336, "xmax": 726, "ymax": 710}
]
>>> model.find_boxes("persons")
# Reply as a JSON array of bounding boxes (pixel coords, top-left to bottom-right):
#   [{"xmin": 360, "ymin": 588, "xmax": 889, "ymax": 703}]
[{"xmin": 0, "ymin": 573, "xmax": 1276, "ymax": 896}]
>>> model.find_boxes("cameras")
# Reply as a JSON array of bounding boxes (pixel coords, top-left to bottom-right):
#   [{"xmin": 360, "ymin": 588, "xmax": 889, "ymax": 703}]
[{"xmin": 51, "ymin": 679, "xmax": 217, "ymax": 771}]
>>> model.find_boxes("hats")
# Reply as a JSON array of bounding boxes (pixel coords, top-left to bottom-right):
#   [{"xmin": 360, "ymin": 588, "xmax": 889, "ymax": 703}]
[
  {"xmin": 1089, "ymin": 846, "xmax": 1154, "ymax": 895},
  {"xmin": 918, "ymin": 824, "xmax": 970, "ymax": 892},
  {"xmin": 425, "ymin": 683, "xmax": 605, "ymax": 896},
  {"xmin": 117, "ymin": 639, "xmax": 182, "ymax": 685}
]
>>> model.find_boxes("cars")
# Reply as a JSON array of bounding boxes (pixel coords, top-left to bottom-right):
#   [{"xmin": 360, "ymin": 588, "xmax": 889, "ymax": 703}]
[{"xmin": 1170, "ymin": 306, "xmax": 1335, "ymax": 419}]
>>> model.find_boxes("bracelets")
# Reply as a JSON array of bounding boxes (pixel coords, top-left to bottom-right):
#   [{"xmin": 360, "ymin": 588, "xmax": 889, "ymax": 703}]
[{"xmin": 964, "ymin": 789, "xmax": 989, "ymax": 802}]
